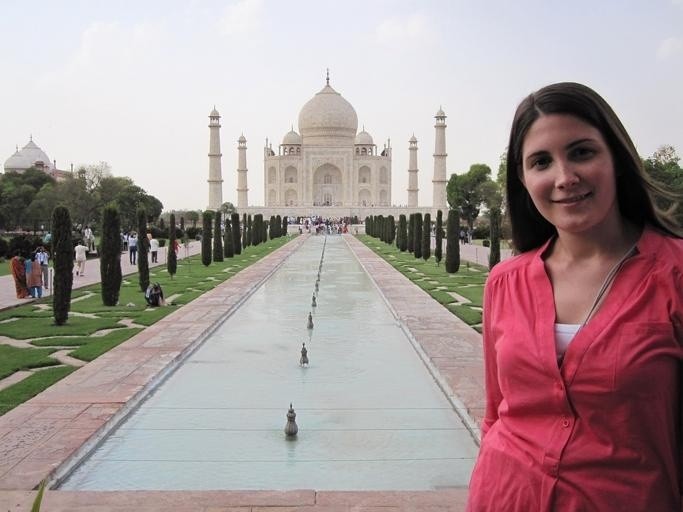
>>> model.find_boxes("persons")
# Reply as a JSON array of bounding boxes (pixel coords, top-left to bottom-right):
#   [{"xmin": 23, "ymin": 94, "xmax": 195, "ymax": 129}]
[
  {"xmin": 43, "ymin": 231, "xmax": 50, "ymax": 241},
  {"xmin": 120, "ymin": 228, "xmax": 159, "ymax": 266},
  {"xmin": 466, "ymin": 225, "xmax": 474, "ymax": 248},
  {"xmin": 72, "ymin": 240, "xmax": 89, "ymax": 277},
  {"xmin": 459, "ymin": 79, "xmax": 683, "ymax": 512},
  {"xmin": 8, "ymin": 248, "xmax": 30, "ymax": 299},
  {"xmin": 33, "ymin": 245, "xmax": 51, "ymax": 289},
  {"xmin": 86, "ymin": 232, "xmax": 95, "ymax": 252},
  {"xmin": 459, "ymin": 228, "xmax": 466, "ymax": 248},
  {"xmin": 286, "ymin": 216, "xmax": 353, "ymax": 235},
  {"xmin": 142, "ymin": 281, "xmax": 164, "ymax": 308},
  {"xmin": 83, "ymin": 226, "xmax": 92, "ymax": 238},
  {"xmin": 24, "ymin": 252, "xmax": 43, "ymax": 299},
  {"xmin": 173, "ymin": 240, "xmax": 181, "ymax": 258}
]
